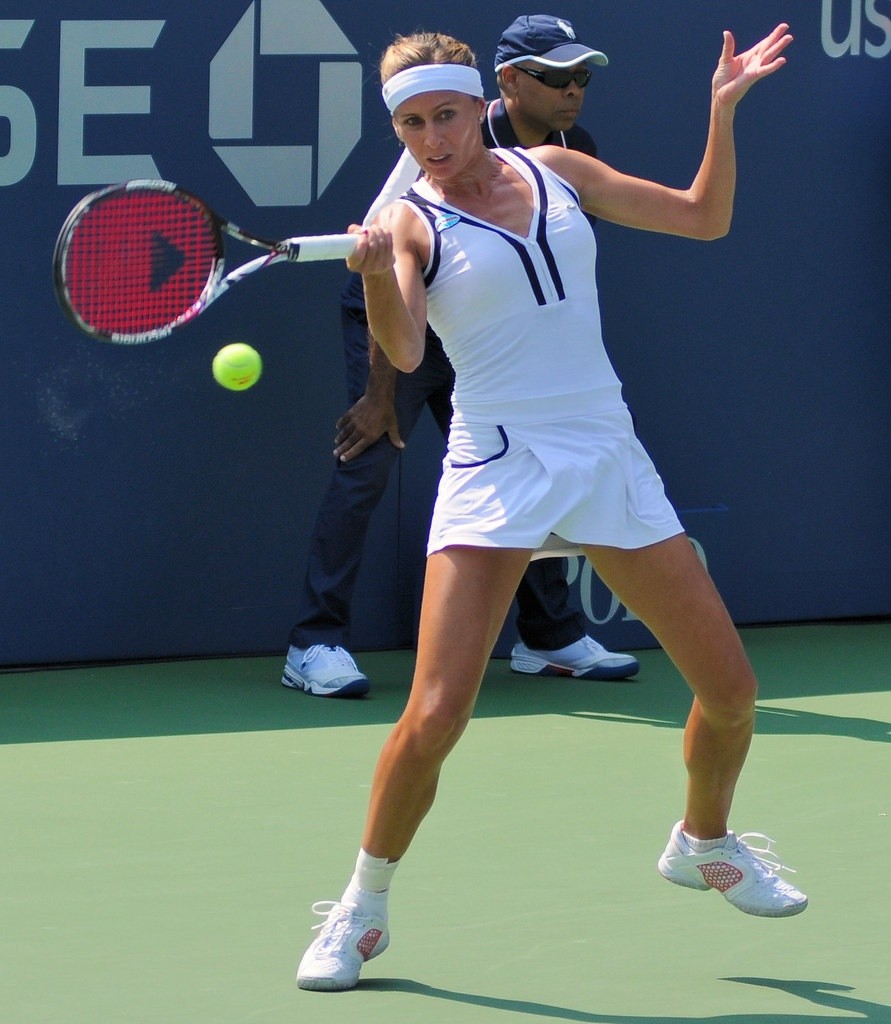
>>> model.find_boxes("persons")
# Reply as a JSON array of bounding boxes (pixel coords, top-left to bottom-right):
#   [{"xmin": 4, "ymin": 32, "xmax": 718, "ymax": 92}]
[
  {"xmin": 283, "ymin": 14, "xmax": 643, "ymax": 701},
  {"xmin": 287, "ymin": 22, "xmax": 812, "ymax": 992}
]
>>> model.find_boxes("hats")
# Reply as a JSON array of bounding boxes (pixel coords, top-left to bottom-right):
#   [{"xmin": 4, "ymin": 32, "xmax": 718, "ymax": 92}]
[{"xmin": 494, "ymin": 15, "xmax": 609, "ymax": 72}]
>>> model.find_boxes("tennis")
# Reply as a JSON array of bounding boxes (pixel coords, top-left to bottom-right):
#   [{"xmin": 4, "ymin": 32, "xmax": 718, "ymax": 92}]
[{"xmin": 212, "ymin": 341, "xmax": 263, "ymax": 393}]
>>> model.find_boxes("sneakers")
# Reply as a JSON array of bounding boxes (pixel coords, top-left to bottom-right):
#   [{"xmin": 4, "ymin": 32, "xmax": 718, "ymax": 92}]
[
  {"xmin": 296, "ymin": 901, "xmax": 390, "ymax": 990},
  {"xmin": 510, "ymin": 635, "xmax": 639, "ymax": 680},
  {"xmin": 658, "ymin": 820, "xmax": 808, "ymax": 918},
  {"xmin": 281, "ymin": 644, "xmax": 370, "ymax": 696}
]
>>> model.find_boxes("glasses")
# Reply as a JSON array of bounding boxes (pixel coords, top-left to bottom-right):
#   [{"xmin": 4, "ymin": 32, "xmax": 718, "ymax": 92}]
[{"xmin": 511, "ymin": 64, "xmax": 593, "ymax": 89}]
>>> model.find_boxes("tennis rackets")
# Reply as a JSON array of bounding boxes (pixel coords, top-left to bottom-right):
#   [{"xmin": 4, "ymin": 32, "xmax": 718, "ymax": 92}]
[{"xmin": 52, "ymin": 179, "xmax": 363, "ymax": 349}]
